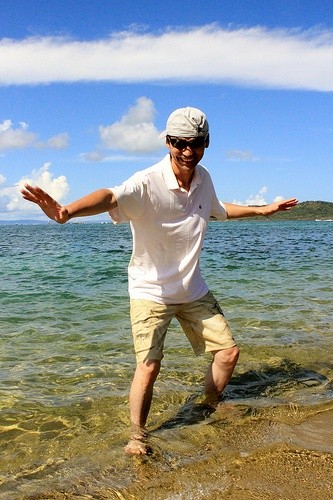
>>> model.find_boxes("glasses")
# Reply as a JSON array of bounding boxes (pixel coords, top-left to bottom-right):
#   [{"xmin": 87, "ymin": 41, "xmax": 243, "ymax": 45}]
[{"xmin": 167, "ymin": 135, "xmax": 205, "ymax": 148}]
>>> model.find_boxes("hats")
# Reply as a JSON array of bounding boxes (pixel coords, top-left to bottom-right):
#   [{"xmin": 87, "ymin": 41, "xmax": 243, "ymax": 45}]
[{"xmin": 158, "ymin": 106, "xmax": 209, "ymax": 141}]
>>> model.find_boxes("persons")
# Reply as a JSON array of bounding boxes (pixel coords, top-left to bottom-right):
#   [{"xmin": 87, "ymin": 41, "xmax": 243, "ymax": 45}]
[{"xmin": 18, "ymin": 106, "xmax": 299, "ymax": 456}]
